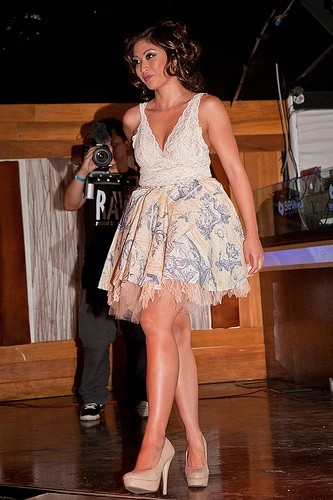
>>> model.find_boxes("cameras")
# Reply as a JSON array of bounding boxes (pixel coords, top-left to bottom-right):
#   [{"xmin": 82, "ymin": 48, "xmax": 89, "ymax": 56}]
[{"xmin": 91, "ymin": 147, "xmax": 112, "ymax": 169}]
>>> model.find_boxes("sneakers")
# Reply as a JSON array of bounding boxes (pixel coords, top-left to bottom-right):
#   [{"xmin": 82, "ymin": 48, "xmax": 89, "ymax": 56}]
[
  {"xmin": 80, "ymin": 403, "xmax": 102, "ymax": 421},
  {"xmin": 135, "ymin": 401, "xmax": 149, "ymax": 417}
]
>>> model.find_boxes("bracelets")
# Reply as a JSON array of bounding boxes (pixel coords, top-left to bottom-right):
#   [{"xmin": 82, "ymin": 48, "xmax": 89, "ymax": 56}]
[{"xmin": 74, "ymin": 175, "xmax": 86, "ymax": 182}]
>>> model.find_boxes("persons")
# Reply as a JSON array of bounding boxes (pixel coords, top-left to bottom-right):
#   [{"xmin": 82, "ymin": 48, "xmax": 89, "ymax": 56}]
[
  {"xmin": 97, "ymin": 19, "xmax": 265, "ymax": 494},
  {"xmin": 63, "ymin": 118, "xmax": 151, "ymax": 426}
]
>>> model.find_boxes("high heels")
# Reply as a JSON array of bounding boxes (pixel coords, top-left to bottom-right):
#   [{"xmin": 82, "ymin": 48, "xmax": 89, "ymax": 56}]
[
  {"xmin": 185, "ymin": 435, "xmax": 209, "ymax": 487},
  {"xmin": 123, "ymin": 438, "xmax": 175, "ymax": 496}
]
